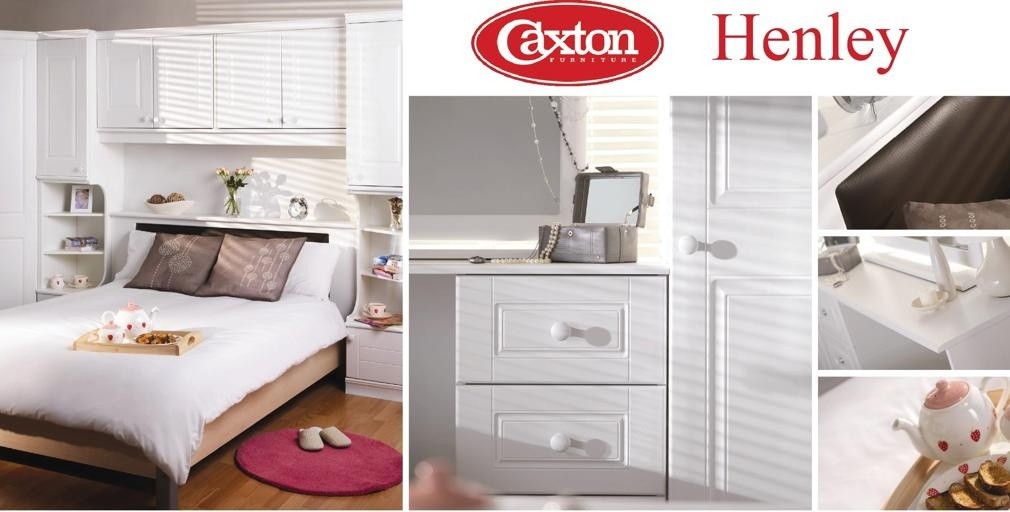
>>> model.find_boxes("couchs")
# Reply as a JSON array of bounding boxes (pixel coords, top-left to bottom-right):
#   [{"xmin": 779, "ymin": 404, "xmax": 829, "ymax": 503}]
[{"xmin": 816, "ymin": 95, "xmax": 1010, "ymax": 230}]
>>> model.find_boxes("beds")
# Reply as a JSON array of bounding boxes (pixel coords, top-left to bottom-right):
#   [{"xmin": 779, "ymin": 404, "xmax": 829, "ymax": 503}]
[{"xmin": 0, "ymin": 222, "xmax": 346, "ymax": 511}]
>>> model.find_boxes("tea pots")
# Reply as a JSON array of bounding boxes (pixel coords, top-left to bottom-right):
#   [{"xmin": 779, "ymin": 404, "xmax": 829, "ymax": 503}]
[
  {"xmin": 99, "ymin": 300, "xmax": 163, "ymax": 342},
  {"xmin": 886, "ymin": 369, "xmax": 1010, "ymax": 465}
]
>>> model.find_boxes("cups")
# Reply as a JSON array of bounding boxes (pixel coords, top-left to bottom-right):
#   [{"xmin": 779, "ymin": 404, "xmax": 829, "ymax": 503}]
[
  {"xmin": 99, "ymin": 318, "xmax": 126, "ymax": 344},
  {"xmin": 70, "ymin": 274, "xmax": 89, "ymax": 288},
  {"xmin": 362, "ymin": 302, "xmax": 387, "ymax": 316}
]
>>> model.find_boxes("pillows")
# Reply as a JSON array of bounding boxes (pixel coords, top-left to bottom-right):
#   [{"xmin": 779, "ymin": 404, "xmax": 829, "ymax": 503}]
[
  {"xmin": 192, "ymin": 232, "xmax": 308, "ymax": 302},
  {"xmin": 123, "ymin": 231, "xmax": 224, "ymax": 296},
  {"xmin": 903, "ymin": 199, "xmax": 1010, "ymax": 230}
]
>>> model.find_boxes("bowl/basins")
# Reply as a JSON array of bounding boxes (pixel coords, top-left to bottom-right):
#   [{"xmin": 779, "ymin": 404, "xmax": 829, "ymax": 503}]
[{"xmin": 144, "ymin": 198, "xmax": 196, "ymax": 217}]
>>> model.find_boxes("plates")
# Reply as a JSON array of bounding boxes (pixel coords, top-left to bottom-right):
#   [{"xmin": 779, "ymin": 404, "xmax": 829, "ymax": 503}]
[
  {"xmin": 134, "ymin": 330, "xmax": 182, "ymax": 346},
  {"xmin": 907, "ymin": 452, "xmax": 1008, "ymax": 512},
  {"xmin": 362, "ymin": 315, "xmax": 393, "ymax": 320}
]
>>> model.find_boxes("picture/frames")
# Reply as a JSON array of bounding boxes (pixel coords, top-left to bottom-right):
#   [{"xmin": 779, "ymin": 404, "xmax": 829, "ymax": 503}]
[{"xmin": 70, "ymin": 185, "xmax": 93, "ymax": 214}]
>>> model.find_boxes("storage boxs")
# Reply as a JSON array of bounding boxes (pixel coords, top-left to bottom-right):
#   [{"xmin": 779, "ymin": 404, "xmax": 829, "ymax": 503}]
[{"xmin": 539, "ymin": 167, "xmax": 652, "ymax": 264}]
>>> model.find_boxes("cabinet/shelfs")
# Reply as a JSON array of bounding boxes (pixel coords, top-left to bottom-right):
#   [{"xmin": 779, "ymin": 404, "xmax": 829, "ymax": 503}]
[
  {"xmin": 97, "ymin": 19, "xmax": 355, "ymax": 227},
  {"xmin": 345, "ymin": 11, "xmax": 405, "ymax": 404},
  {"xmin": 0, "ymin": 29, "xmax": 113, "ymax": 311},
  {"xmin": 408, "ymin": 261, "xmax": 667, "ymax": 512},
  {"xmin": 818, "ymin": 261, "xmax": 1010, "ymax": 370}
]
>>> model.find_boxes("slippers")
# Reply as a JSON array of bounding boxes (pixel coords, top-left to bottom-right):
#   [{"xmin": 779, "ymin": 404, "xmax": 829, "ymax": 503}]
[
  {"xmin": 316, "ymin": 426, "xmax": 353, "ymax": 448},
  {"xmin": 299, "ymin": 427, "xmax": 324, "ymax": 450}
]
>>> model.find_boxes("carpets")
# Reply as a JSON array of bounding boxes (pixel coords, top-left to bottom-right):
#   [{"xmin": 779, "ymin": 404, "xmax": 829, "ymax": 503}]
[{"xmin": 235, "ymin": 428, "xmax": 402, "ymax": 496}]
